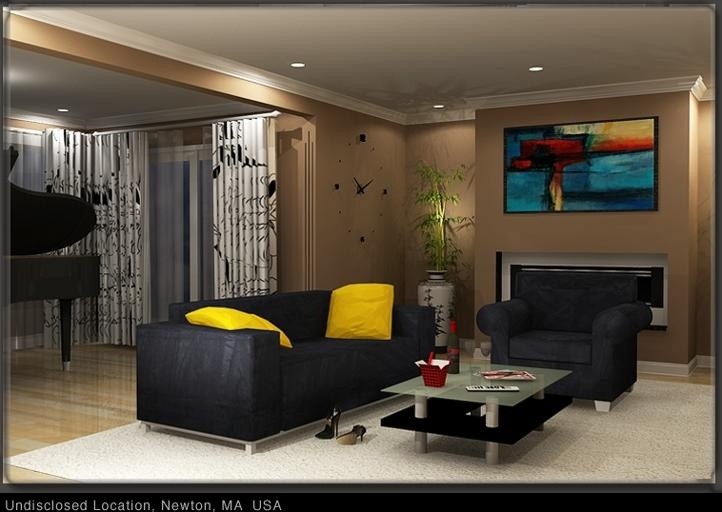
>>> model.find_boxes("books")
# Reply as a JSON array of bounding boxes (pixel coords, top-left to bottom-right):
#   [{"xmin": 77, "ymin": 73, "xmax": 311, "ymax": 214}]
[{"xmin": 479, "ymin": 368, "xmax": 536, "ymax": 381}]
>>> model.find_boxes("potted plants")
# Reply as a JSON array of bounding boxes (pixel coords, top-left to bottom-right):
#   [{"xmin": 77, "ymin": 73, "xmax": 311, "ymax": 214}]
[{"xmin": 402, "ymin": 158, "xmax": 475, "ymax": 354}]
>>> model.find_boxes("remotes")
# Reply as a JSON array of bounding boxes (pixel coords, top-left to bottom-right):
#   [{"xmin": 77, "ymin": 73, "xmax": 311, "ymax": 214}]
[{"xmin": 465, "ymin": 384, "xmax": 520, "ymax": 392}]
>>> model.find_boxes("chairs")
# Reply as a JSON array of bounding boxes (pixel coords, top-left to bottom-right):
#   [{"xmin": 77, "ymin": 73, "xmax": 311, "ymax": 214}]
[{"xmin": 476, "ymin": 270, "xmax": 653, "ymax": 413}]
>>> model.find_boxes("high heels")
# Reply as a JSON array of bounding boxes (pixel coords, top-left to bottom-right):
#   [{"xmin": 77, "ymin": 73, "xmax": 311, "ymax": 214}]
[
  {"xmin": 315, "ymin": 405, "xmax": 342, "ymax": 440},
  {"xmin": 336, "ymin": 424, "xmax": 366, "ymax": 445}
]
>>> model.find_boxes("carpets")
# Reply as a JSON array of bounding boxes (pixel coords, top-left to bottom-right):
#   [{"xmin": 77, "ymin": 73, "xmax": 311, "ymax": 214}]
[{"xmin": 1, "ymin": 377, "xmax": 717, "ymax": 486}]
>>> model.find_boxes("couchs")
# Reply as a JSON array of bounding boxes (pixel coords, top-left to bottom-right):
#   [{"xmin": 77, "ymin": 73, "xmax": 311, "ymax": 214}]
[{"xmin": 136, "ymin": 290, "xmax": 435, "ymax": 454}]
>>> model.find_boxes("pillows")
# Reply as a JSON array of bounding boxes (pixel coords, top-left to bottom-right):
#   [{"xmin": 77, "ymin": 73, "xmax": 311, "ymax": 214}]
[
  {"xmin": 184, "ymin": 306, "xmax": 294, "ymax": 349},
  {"xmin": 325, "ymin": 283, "xmax": 394, "ymax": 341}
]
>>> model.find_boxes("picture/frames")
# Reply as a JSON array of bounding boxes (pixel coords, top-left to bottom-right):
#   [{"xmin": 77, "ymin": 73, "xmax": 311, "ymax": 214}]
[{"xmin": 503, "ymin": 116, "xmax": 658, "ymax": 213}]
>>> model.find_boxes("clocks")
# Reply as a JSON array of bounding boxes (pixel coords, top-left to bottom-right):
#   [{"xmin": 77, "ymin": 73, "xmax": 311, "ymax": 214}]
[{"xmin": 331, "ymin": 130, "xmax": 388, "ymax": 244}]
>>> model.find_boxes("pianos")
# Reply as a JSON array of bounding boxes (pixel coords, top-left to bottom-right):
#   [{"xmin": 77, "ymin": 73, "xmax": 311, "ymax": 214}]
[{"xmin": 0, "ymin": 145, "xmax": 101, "ymax": 372}]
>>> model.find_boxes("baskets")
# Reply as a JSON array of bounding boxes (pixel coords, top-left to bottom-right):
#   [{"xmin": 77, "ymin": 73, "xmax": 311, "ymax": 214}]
[{"xmin": 418, "ymin": 350, "xmax": 451, "ymax": 387}]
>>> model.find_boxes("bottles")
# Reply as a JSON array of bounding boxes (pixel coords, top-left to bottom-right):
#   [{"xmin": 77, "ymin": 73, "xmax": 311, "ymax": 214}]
[{"xmin": 447, "ymin": 323, "xmax": 459, "ymax": 373}]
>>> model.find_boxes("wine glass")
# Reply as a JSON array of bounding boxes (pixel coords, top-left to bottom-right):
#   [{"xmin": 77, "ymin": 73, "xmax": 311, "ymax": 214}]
[
  {"xmin": 462, "ymin": 339, "xmax": 477, "ymax": 373},
  {"xmin": 479, "ymin": 342, "xmax": 492, "ymax": 373}
]
